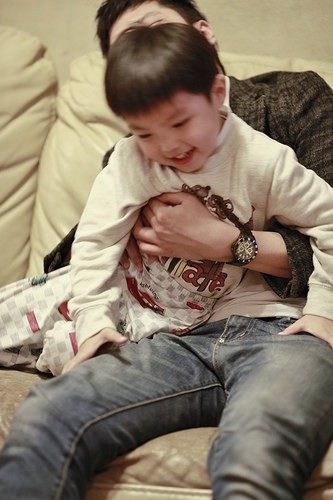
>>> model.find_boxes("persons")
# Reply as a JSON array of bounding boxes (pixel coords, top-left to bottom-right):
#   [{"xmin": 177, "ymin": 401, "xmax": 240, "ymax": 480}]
[
  {"xmin": 0, "ymin": 20, "xmax": 333, "ymax": 376},
  {"xmin": 0, "ymin": 0, "xmax": 333, "ymax": 500}
]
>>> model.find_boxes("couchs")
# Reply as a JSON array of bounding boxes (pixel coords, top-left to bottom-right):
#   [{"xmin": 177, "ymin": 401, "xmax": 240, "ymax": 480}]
[{"xmin": 0, "ymin": 25, "xmax": 333, "ymax": 500}]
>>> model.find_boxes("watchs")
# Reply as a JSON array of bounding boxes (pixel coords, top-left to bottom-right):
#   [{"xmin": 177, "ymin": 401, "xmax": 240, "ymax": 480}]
[{"xmin": 225, "ymin": 226, "xmax": 259, "ymax": 268}]
